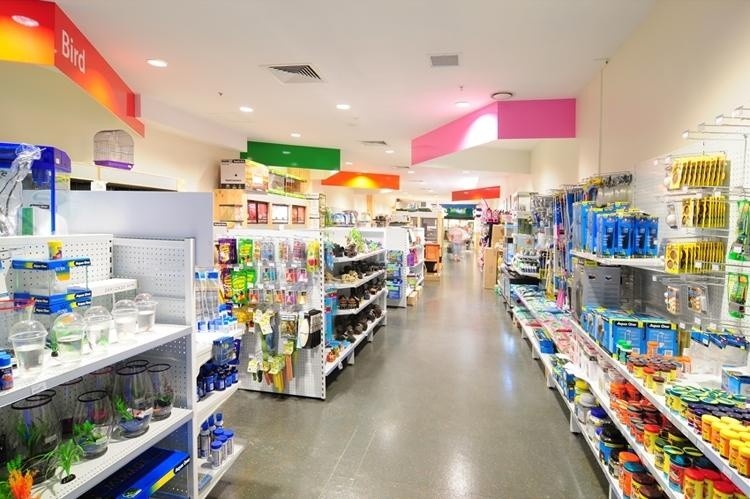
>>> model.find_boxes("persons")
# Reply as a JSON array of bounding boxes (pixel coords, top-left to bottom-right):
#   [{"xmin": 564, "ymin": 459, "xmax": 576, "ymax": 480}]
[{"xmin": 444, "ymin": 223, "xmax": 472, "ymax": 262}]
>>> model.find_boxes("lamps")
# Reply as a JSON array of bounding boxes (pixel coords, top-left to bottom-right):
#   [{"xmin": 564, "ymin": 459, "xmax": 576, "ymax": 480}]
[{"xmin": 492, "ymin": 92, "xmax": 512, "ymax": 100}]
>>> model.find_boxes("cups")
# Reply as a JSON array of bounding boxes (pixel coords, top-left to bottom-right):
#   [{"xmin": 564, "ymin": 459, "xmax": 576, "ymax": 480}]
[
  {"xmin": 52, "ymin": 312, "xmax": 86, "ymax": 364},
  {"xmin": 9, "ymin": 320, "xmax": 48, "ymax": 375},
  {"xmin": 111, "ymin": 300, "xmax": 139, "ymax": 342},
  {"xmin": 133, "ymin": 292, "xmax": 158, "ymax": 335},
  {"xmin": 83, "ymin": 306, "xmax": 113, "ymax": 353}
]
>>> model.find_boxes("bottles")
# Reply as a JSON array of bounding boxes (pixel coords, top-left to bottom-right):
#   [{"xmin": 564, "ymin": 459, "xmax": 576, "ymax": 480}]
[
  {"xmin": 3, "ymin": 357, "xmax": 176, "ymax": 487},
  {"xmin": 197, "ymin": 413, "xmax": 235, "ymax": 468},
  {"xmin": 195, "ymin": 359, "xmax": 240, "ymax": 403}
]
